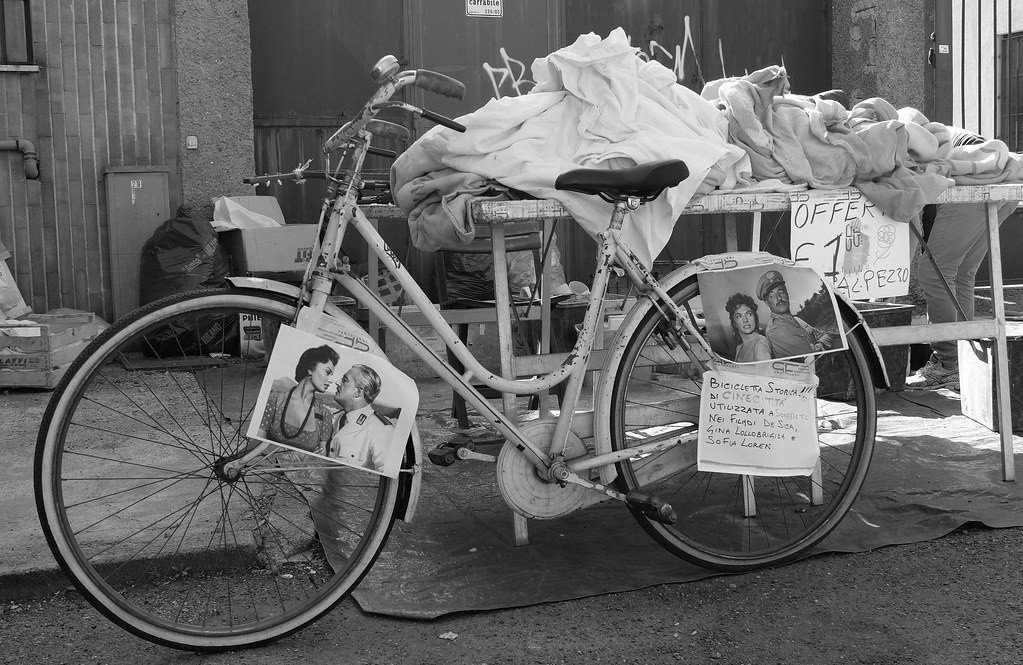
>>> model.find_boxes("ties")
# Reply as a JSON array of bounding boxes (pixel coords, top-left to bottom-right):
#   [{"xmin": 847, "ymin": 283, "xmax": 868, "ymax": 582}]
[{"xmin": 339, "ymin": 412, "xmax": 348, "ymax": 429}]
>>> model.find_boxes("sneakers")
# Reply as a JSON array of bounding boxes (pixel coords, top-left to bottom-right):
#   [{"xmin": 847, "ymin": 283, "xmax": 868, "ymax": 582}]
[{"xmin": 906, "ymin": 355, "xmax": 961, "ymax": 390}]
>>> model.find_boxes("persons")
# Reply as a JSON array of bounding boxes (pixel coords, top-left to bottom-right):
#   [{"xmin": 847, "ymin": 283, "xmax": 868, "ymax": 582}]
[
  {"xmin": 313, "ymin": 364, "xmax": 396, "ymax": 472},
  {"xmin": 905, "ymin": 126, "xmax": 1020, "ymax": 390},
  {"xmin": 725, "ymin": 292, "xmax": 771, "ymax": 363},
  {"xmin": 256, "ymin": 344, "xmax": 340, "ymax": 456},
  {"xmin": 756, "ymin": 270, "xmax": 833, "ymax": 359}
]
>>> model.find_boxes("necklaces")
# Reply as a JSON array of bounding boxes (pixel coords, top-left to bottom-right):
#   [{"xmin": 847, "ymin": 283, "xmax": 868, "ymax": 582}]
[{"xmin": 279, "ymin": 385, "xmax": 316, "ymax": 440}]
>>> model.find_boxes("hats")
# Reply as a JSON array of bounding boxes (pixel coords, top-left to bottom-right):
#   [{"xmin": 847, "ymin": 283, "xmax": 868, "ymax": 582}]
[{"xmin": 756, "ymin": 270, "xmax": 786, "ymax": 300}]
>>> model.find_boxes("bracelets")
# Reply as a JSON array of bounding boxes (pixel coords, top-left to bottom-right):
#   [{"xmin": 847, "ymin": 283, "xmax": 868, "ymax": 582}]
[{"xmin": 814, "ymin": 345, "xmax": 820, "ymax": 349}]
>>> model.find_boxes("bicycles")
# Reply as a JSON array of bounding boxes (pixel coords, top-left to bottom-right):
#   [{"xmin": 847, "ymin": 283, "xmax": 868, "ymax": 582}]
[{"xmin": 34, "ymin": 55, "xmax": 891, "ymax": 653}]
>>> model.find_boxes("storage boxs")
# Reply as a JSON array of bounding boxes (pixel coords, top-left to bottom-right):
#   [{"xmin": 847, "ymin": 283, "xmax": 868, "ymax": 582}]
[
  {"xmin": 841, "ymin": 298, "xmax": 919, "ymax": 394},
  {"xmin": 1, "ymin": 314, "xmax": 98, "ymax": 392},
  {"xmin": 212, "ymin": 193, "xmax": 321, "ymax": 272},
  {"xmin": 553, "ymin": 294, "xmax": 654, "ymax": 383}
]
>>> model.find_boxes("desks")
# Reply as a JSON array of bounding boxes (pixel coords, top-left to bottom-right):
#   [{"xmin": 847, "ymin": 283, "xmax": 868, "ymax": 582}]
[{"xmin": 357, "ymin": 183, "xmax": 1023, "ymax": 545}]
